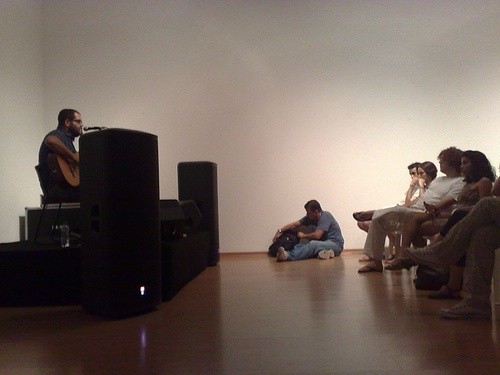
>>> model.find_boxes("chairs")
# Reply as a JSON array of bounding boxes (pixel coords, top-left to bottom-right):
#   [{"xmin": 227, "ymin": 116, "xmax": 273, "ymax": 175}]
[{"xmin": 34, "ymin": 166, "xmax": 80, "ymax": 242}]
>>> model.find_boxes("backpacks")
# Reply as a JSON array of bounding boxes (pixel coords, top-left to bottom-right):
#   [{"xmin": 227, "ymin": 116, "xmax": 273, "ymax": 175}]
[{"xmin": 268, "ymin": 230, "xmax": 301, "ymax": 257}]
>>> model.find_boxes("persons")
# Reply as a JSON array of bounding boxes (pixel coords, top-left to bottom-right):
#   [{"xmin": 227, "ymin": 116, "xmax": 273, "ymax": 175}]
[
  {"xmin": 353, "ymin": 147, "xmax": 500, "ymax": 319},
  {"xmin": 273, "ymin": 200, "xmax": 344, "ymax": 262},
  {"xmin": 39, "ymin": 108, "xmax": 84, "ymax": 203}
]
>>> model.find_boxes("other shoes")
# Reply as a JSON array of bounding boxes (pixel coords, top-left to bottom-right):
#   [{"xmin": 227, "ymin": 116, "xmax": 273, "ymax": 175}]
[
  {"xmin": 384, "ymin": 256, "xmax": 417, "ymax": 271},
  {"xmin": 356, "ymin": 266, "xmax": 382, "ymax": 274},
  {"xmin": 318, "ymin": 249, "xmax": 334, "ymax": 259},
  {"xmin": 277, "ymin": 247, "xmax": 288, "ymax": 261},
  {"xmin": 428, "ymin": 284, "xmax": 462, "ymax": 300},
  {"xmin": 357, "ymin": 221, "xmax": 368, "ymax": 232},
  {"xmin": 352, "ymin": 211, "xmax": 372, "ymax": 221}
]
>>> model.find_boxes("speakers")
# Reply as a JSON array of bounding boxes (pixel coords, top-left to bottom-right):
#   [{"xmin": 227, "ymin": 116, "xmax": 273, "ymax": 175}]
[{"xmin": 79, "ymin": 128, "xmax": 220, "ymax": 321}]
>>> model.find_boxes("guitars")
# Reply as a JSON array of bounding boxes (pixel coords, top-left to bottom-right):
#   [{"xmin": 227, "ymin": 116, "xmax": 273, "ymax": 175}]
[{"xmin": 45, "ymin": 149, "xmax": 81, "ymax": 187}]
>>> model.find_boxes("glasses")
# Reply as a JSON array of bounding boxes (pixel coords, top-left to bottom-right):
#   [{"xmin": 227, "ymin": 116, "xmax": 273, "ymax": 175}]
[{"xmin": 69, "ymin": 119, "xmax": 82, "ymax": 124}]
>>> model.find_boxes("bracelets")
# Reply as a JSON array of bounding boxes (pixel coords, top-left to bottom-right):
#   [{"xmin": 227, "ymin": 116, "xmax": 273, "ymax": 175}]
[{"xmin": 278, "ymin": 228, "xmax": 284, "ymax": 234}]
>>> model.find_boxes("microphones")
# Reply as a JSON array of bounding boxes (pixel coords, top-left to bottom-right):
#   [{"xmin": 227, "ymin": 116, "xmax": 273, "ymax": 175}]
[{"xmin": 84, "ymin": 126, "xmax": 103, "ymax": 130}]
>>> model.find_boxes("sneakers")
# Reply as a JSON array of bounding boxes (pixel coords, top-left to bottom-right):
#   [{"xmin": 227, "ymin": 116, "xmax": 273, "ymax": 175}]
[
  {"xmin": 439, "ymin": 295, "xmax": 492, "ymax": 319},
  {"xmin": 404, "ymin": 243, "xmax": 451, "ymax": 274}
]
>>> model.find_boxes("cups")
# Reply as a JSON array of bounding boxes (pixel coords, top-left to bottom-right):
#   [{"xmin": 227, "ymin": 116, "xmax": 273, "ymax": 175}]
[{"xmin": 61, "ymin": 225, "xmax": 69, "ymax": 248}]
